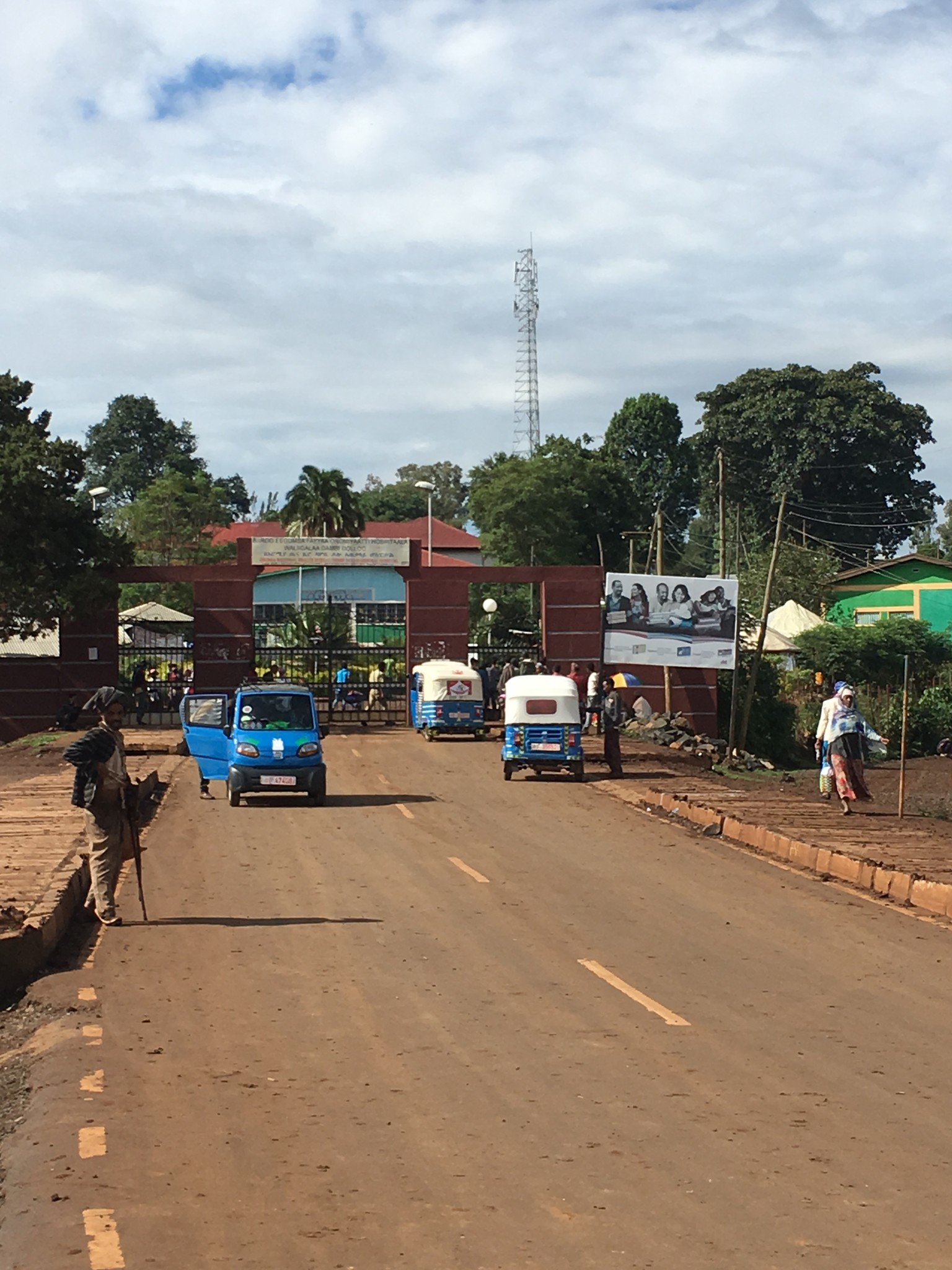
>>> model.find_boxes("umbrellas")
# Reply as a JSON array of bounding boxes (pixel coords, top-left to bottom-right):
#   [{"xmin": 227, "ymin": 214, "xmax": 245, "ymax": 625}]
[{"xmin": 609, "ymin": 672, "xmax": 642, "ymax": 689}]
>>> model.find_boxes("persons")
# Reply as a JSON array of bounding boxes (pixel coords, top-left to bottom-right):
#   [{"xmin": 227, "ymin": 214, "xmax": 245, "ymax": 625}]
[
  {"xmin": 603, "ymin": 580, "xmax": 736, "ymax": 639},
  {"xmin": 813, "ymin": 681, "xmax": 889, "ymax": 817},
  {"xmin": 189, "ymin": 694, "xmax": 234, "ymax": 799},
  {"xmin": 470, "ymin": 654, "xmax": 653, "ymax": 737},
  {"xmin": 119, "ymin": 659, "xmax": 397, "ymax": 726},
  {"xmin": 578, "ymin": 678, "xmax": 623, "ymax": 779},
  {"xmin": 61, "ymin": 687, "xmax": 132, "ymax": 926}
]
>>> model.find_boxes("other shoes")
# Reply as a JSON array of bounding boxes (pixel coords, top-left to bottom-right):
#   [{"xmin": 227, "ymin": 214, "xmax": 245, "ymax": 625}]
[
  {"xmin": 607, "ymin": 772, "xmax": 624, "ymax": 779},
  {"xmin": 94, "ymin": 908, "xmax": 122, "ymax": 926},
  {"xmin": 822, "ymin": 792, "xmax": 831, "ymax": 799},
  {"xmin": 200, "ymin": 791, "xmax": 214, "ymax": 799}
]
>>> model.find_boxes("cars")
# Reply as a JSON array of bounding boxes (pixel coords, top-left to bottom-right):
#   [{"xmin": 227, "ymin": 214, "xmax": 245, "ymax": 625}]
[{"xmin": 178, "ymin": 683, "xmax": 330, "ymax": 807}]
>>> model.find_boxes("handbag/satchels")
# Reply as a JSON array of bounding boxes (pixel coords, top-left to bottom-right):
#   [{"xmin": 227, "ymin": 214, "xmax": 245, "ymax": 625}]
[{"xmin": 820, "ymin": 762, "xmax": 837, "ymax": 792}]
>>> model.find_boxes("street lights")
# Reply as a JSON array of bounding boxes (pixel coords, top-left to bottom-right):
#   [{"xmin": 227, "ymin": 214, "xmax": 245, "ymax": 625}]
[
  {"xmin": 414, "ymin": 481, "xmax": 436, "ymax": 568},
  {"xmin": 482, "ymin": 598, "xmax": 497, "ymax": 646},
  {"xmin": 88, "ymin": 486, "xmax": 110, "ymax": 525}
]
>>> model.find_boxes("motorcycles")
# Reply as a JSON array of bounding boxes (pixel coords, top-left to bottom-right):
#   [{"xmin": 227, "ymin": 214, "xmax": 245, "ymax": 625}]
[
  {"xmin": 406, "ymin": 661, "xmax": 485, "ymax": 742},
  {"xmin": 501, "ymin": 675, "xmax": 584, "ymax": 783}
]
